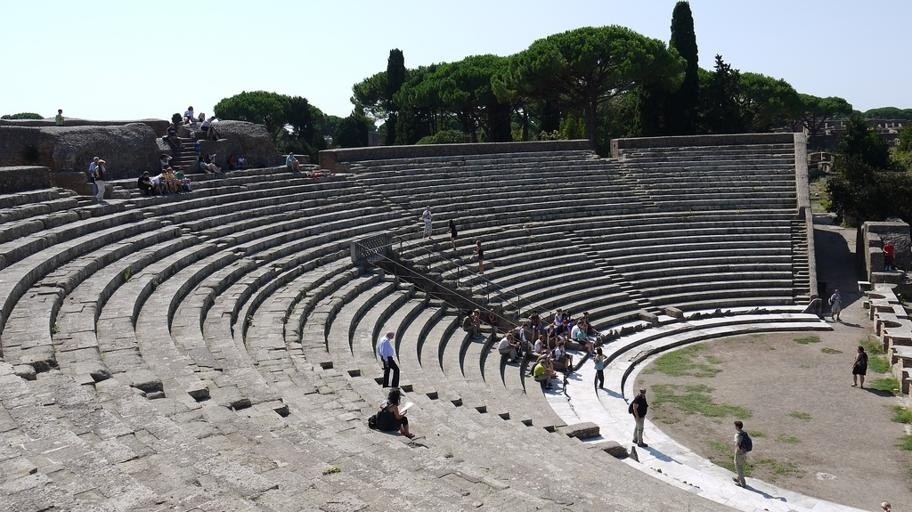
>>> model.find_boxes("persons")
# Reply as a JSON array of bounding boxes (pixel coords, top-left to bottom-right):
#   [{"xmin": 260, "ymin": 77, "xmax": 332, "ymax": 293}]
[
  {"xmin": 731, "ymin": 420, "xmax": 747, "ymax": 487},
  {"xmin": 89, "ymin": 157, "xmax": 100, "ymax": 184},
  {"xmin": 374, "ymin": 388, "xmax": 416, "ymax": 439},
  {"xmin": 92, "ymin": 159, "xmax": 107, "ymax": 201},
  {"xmin": 830, "ymin": 288, "xmax": 842, "ymax": 322},
  {"xmin": 376, "ymin": 331, "xmax": 400, "ymax": 388},
  {"xmin": 286, "ymin": 151, "xmax": 301, "ymax": 173},
  {"xmin": 470, "ymin": 239, "xmax": 485, "ymax": 274},
  {"xmin": 461, "ymin": 307, "xmax": 608, "ymax": 390},
  {"xmin": 447, "ymin": 218, "xmax": 458, "ymax": 250},
  {"xmin": 136, "ymin": 106, "xmax": 248, "ymax": 195},
  {"xmin": 883, "ymin": 241, "xmax": 895, "ymax": 271},
  {"xmin": 627, "ymin": 388, "xmax": 649, "ymax": 448},
  {"xmin": 421, "ymin": 205, "xmax": 432, "ymax": 240},
  {"xmin": 55, "ymin": 109, "xmax": 64, "ymax": 126},
  {"xmin": 850, "ymin": 346, "xmax": 868, "ymax": 390}
]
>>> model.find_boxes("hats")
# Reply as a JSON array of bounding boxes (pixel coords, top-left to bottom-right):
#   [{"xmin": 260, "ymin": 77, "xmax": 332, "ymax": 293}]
[{"xmin": 389, "ymin": 389, "xmax": 405, "ymax": 397}]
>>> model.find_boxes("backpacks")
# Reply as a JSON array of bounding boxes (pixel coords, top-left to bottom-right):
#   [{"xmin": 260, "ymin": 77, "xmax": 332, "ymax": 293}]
[
  {"xmin": 628, "ymin": 393, "xmax": 639, "ymax": 413},
  {"xmin": 737, "ymin": 432, "xmax": 752, "ymax": 451}
]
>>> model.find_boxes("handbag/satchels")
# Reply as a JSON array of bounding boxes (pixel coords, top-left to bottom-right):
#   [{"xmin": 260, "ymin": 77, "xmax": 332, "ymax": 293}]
[{"xmin": 367, "ymin": 414, "xmax": 378, "ymax": 430}]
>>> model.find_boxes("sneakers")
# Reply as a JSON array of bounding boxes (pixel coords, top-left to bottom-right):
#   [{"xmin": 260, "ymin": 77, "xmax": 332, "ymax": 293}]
[
  {"xmin": 732, "ymin": 476, "xmax": 745, "ymax": 488},
  {"xmin": 633, "ymin": 440, "xmax": 649, "ymax": 446}
]
